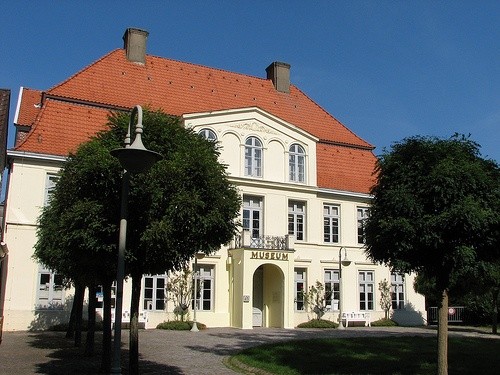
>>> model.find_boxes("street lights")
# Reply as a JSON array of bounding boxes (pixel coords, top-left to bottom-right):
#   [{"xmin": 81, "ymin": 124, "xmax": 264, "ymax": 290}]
[
  {"xmin": 188, "ymin": 244, "xmax": 206, "ymax": 333},
  {"xmin": 336, "ymin": 246, "xmax": 353, "ymax": 329},
  {"xmin": 110, "ymin": 103, "xmax": 164, "ymax": 374}
]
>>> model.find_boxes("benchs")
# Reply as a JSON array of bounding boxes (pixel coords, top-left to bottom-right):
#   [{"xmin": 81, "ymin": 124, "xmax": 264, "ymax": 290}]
[
  {"xmin": 337, "ymin": 311, "xmax": 373, "ymax": 328},
  {"xmin": 111, "ymin": 322, "xmax": 146, "ymax": 330}
]
